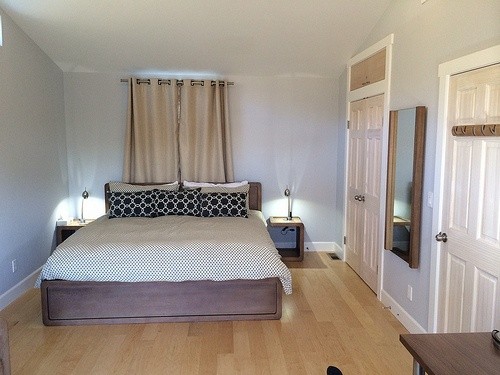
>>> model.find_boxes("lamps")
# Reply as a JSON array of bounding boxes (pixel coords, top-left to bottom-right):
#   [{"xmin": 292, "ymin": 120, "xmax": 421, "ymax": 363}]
[
  {"xmin": 284, "ymin": 185, "xmax": 292, "ymax": 220},
  {"xmin": 79, "ymin": 187, "xmax": 88, "ymax": 223}
]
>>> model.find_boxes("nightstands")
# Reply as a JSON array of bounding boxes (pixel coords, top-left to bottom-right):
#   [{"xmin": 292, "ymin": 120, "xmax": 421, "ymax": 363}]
[
  {"xmin": 267, "ymin": 216, "xmax": 305, "ymax": 262},
  {"xmin": 57, "ymin": 219, "xmax": 94, "ymax": 245}
]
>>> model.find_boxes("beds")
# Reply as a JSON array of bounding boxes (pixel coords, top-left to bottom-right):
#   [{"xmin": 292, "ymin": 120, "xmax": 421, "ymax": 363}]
[{"xmin": 34, "ymin": 183, "xmax": 292, "ymax": 326}]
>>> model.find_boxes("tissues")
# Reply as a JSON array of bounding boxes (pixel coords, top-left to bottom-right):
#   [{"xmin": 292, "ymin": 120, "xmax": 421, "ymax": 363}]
[{"xmin": 56, "ymin": 210, "xmax": 74, "ymax": 226}]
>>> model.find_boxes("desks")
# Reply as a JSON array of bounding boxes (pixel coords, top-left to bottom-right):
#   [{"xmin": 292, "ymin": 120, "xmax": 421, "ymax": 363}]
[{"xmin": 399, "ymin": 332, "xmax": 500, "ymax": 375}]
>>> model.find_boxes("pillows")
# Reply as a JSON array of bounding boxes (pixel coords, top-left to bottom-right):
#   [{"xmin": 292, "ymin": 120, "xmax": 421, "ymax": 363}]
[
  {"xmin": 108, "ymin": 180, "xmax": 179, "ymax": 192},
  {"xmin": 106, "ymin": 189, "xmax": 159, "ymax": 219},
  {"xmin": 156, "ymin": 187, "xmax": 202, "ymax": 217},
  {"xmin": 201, "ymin": 192, "xmax": 248, "ymax": 218},
  {"xmin": 183, "ymin": 179, "xmax": 248, "ymax": 187},
  {"xmin": 181, "ymin": 184, "xmax": 251, "ymax": 215}
]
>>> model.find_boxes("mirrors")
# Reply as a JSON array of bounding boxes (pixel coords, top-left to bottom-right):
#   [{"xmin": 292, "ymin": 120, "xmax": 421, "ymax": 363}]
[{"xmin": 384, "ymin": 106, "xmax": 426, "ymax": 269}]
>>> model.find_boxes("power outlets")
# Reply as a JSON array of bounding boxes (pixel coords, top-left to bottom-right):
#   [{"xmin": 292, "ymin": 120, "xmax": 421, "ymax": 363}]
[{"xmin": 12, "ymin": 257, "xmax": 17, "ymax": 272}]
